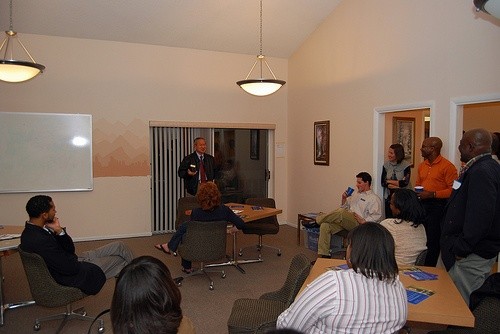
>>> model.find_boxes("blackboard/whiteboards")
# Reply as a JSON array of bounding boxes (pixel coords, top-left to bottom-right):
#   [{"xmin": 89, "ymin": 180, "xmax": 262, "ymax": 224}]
[{"xmin": 0, "ymin": 112, "xmax": 93, "ymax": 193}]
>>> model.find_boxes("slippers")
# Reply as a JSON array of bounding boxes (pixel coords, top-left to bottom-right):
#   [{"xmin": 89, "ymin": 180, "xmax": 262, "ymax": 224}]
[
  {"xmin": 181, "ymin": 268, "xmax": 191, "ymax": 274},
  {"xmin": 153, "ymin": 243, "xmax": 171, "ymax": 255}
]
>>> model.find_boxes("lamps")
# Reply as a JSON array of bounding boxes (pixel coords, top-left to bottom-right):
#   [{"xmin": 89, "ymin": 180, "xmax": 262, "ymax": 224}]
[
  {"xmin": 236, "ymin": 0, "xmax": 286, "ymax": 96},
  {"xmin": 0, "ymin": 0, "xmax": 46, "ymax": 83}
]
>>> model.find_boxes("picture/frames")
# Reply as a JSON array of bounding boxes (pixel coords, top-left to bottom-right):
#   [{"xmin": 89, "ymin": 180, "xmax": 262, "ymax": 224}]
[
  {"xmin": 393, "ymin": 117, "xmax": 415, "ymax": 168},
  {"xmin": 314, "ymin": 120, "xmax": 330, "ymax": 166}
]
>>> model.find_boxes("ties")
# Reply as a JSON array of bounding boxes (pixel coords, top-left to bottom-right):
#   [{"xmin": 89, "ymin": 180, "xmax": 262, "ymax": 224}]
[
  {"xmin": 200, "ymin": 155, "xmax": 206, "ymax": 183},
  {"xmin": 45, "ymin": 226, "xmax": 57, "ymax": 239}
]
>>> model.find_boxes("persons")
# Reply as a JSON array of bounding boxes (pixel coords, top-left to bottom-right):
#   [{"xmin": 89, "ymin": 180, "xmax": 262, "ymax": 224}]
[
  {"xmin": 155, "ymin": 182, "xmax": 246, "ymax": 274},
  {"xmin": 178, "ymin": 137, "xmax": 219, "ymax": 197},
  {"xmin": 381, "ymin": 144, "xmax": 411, "ymax": 219},
  {"xmin": 414, "ymin": 137, "xmax": 459, "ymax": 269},
  {"xmin": 276, "ymin": 221, "xmax": 408, "ymax": 334},
  {"xmin": 110, "ymin": 255, "xmax": 197, "ymax": 334},
  {"xmin": 20, "ymin": 195, "xmax": 139, "ymax": 296},
  {"xmin": 380, "ymin": 188, "xmax": 427, "ymax": 267},
  {"xmin": 301, "ymin": 172, "xmax": 384, "ymax": 265},
  {"xmin": 152, "ymin": 139, "xmax": 239, "ymax": 192},
  {"xmin": 423, "ymin": 128, "xmax": 500, "ymax": 307}
]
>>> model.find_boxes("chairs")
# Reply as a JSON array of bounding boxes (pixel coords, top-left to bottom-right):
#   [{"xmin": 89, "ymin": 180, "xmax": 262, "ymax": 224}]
[
  {"xmin": 176, "ymin": 196, "xmax": 311, "ymax": 334},
  {"xmin": 18, "ymin": 244, "xmax": 104, "ymax": 334}
]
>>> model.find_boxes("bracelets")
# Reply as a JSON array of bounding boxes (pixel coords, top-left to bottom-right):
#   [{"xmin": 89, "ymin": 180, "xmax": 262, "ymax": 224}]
[{"xmin": 433, "ymin": 191, "xmax": 436, "ymax": 198}]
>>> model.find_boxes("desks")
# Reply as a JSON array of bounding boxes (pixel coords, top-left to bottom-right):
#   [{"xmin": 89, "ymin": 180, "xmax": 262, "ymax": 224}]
[
  {"xmin": 184, "ymin": 203, "xmax": 283, "ymax": 274},
  {"xmin": 295, "ymin": 258, "xmax": 475, "ymax": 334},
  {"xmin": 0, "ymin": 226, "xmax": 36, "ymax": 327}
]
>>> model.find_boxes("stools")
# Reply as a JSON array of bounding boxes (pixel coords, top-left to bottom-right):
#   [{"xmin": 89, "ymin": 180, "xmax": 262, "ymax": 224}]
[{"xmin": 297, "ymin": 212, "xmax": 325, "ymax": 244}]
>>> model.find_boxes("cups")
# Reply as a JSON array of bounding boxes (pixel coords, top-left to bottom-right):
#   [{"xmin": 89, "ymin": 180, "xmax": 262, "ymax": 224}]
[
  {"xmin": 414, "ymin": 186, "xmax": 424, "ymax": 197},
  {"xmin": 345, "ymin": 187, "xmax": 354, "ymax": 197}
]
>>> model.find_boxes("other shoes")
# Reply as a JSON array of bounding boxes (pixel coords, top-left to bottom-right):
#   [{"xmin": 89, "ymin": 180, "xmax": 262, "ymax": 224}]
[
  {"xmin": 302, "ymin": 219, "xmax": 320, "ymax": 228},
  {"xmin": 174, "ymin": 277, "xmax": 183, "ymax": 284},
  {"xmin": 311, "ymin": 255, "xmax": 330, "ymax": 265}
]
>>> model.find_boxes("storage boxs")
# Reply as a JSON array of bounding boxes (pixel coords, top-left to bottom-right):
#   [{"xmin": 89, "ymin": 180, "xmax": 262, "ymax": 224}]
[{"xmin": 301, "ymin": 227, "xmax": 342, "ymax": 251}]
